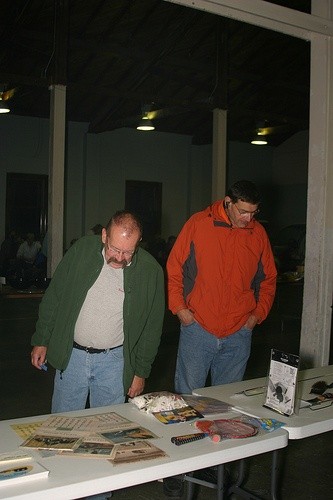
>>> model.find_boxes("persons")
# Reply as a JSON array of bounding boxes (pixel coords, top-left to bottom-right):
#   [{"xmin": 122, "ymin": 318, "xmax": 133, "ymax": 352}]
[
  {"xmin": 31, "ymin": 211, "xmax": 165, "ymax": 415},
  {"xmin": 146, "ymin": 233, "xmax": 175, "ymax": 260},
  {"xmin": 0, "ymin": 228, "xmax": 48, "ymax": 289},
  {"xmin": 167, "ymin": 180, "xmax": 277, "ymax": 393},
  {"xmin": 92, "ymin": 224, "xmax": 102, "ymax": 235},
  {"xmin": 304, "ymin": 392, "xmax": 326, "ymax": 401},
  {"xmin": 115, "ymin": 428, "xmax": 153, "ymax": 439}
]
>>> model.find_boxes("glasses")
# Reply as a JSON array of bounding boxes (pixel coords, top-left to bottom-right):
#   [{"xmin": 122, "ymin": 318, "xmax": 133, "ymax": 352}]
[
  {"xmin": 105, "ymin": 231, "xmax": 138, "ymax": 256},
  {"xmin": 232, "ymin": 200, "xmax": 259, "ymax": 216}
]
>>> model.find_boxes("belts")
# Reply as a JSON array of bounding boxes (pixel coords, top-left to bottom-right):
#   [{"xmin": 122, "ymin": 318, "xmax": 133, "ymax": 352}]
[{"xmin": 72, "ymin": 341, "xmax": 124, "ymax": 353}]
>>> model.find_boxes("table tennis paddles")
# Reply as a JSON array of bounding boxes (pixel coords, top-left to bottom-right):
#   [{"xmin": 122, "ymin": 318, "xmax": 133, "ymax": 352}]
[{"xmin": 196, "ymin": 420, "xmax": 240, "ymax": 442}]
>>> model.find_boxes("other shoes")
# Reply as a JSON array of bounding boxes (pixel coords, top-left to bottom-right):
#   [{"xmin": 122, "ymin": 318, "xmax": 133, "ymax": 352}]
[
  {"xmin": 204, "ymin": 467, "xmax": 229, "ymax": 483},
  {"xmin": 162, "ymin": 474, "xmax": 185, "ymax": 499}
]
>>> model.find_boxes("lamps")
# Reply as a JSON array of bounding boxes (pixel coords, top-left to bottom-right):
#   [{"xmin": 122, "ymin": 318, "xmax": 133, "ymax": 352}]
[
  {"xmin": 0, "ymin": 84, "xmax": 15, "ymax": 113},
  {"xmin": 136, "ymin": 103, "xmax": 156, "ymax": 131},
  {"xmin": 250, "ymin": 122, "xmax": 268, "ymax": 146}
]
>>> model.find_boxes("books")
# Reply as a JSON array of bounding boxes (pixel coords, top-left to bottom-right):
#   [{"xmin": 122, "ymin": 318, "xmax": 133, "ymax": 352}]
[{"xmin": 0, "ymin": 461, "xmax": 49, "ymax": 484}]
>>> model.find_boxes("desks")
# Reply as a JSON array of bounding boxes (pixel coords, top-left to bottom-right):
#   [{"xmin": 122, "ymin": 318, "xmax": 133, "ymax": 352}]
[
  {"xmin": 256, "ymin": 274, "xmax": 304, "ymax": 359},
  {"xmin": 190, "ymin": 364, "xmax": 333, "ymax": 500},
  {"xmin": 0, "ymin": 394, "xmax": 290, "ymax": 500}
]
